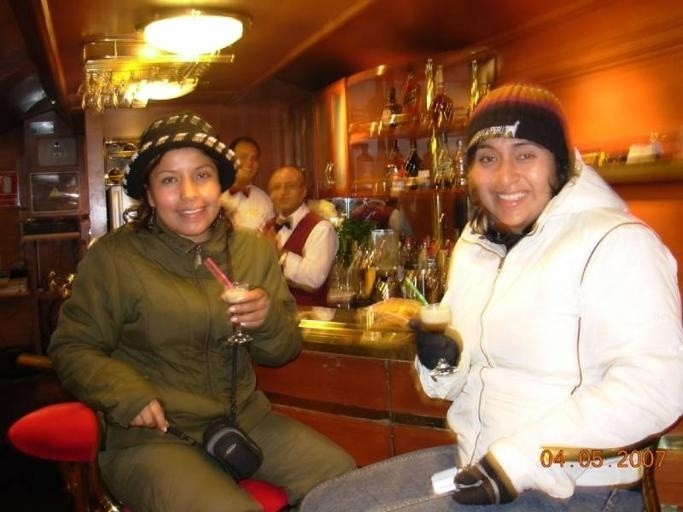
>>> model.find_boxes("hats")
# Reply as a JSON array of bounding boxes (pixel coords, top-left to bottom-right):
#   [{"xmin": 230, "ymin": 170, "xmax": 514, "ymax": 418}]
[
  {"xmin": 465, "ymin": 84, "xmax": 568, "ymax": 164},
  {"xmin": 122, "ymin": 110, "xmax": 240, "ymax": 200}
]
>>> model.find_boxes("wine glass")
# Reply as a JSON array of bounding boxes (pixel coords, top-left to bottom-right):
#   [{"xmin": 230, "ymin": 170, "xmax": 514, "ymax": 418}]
[
  {"xmin": 220, "ymin": 282, "xmax": 254, "ymax": 344},
  {"xmin": 421, "ymin": 304, "xmax": 459, "ymax": 377}
]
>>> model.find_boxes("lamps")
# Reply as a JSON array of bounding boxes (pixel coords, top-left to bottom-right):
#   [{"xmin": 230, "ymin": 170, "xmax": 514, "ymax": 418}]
[{"xmin": 128, "ymin": 8, "xmax": 250, "ymax": 54}]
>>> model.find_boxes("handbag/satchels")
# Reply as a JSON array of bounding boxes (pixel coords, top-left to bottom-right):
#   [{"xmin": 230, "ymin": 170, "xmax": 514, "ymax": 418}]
[{"xmin": 202, "ymin": 419, "xmax": 263, "ymax": 481}]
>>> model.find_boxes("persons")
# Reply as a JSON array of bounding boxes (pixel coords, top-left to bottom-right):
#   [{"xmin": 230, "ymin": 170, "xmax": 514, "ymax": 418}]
[
  {"xmin": 351, "ymin": 200, "xmax": 400, "ymax": 231},
  {"xmin": 299, "ymin": 83, "xmax": 683, "ymax": 512},
  {"xmin": 440, "ymin": 148, "xmax": 453, "ymax": 190},
  {"xmin": 402, "ymin": 75, "xmax": 422, "ymax": 127},
  {"xmin": 430, "ymin": 85, "xmax": 453, "ymax": 130},
  {"xmin": 406, "ymin": 149, "xmax": 423, "ymax": 176}
]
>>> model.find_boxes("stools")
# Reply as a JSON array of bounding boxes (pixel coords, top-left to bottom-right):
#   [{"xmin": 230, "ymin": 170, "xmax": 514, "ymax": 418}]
[{"xmin": 6, "ymin": 401, "xmax": 97, "ymax": 510}]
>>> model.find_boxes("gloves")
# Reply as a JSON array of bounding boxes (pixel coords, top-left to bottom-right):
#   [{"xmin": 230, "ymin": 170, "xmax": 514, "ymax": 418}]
[
  {"xmin": 408, "ymin": 318, "xmax": 458, "ymax": 369},
  {"xmin": 452, "ymin": 451, "xmax": 517, "ymax": 505}
]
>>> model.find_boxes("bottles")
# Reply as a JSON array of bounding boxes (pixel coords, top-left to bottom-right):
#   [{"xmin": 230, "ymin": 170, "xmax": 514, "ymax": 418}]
[
  {"xmin": 327, "ymin": 228, "xmax": 454, "ymax": 308},
  {"xmin": 352, "ymin": 66, "xmax": 467, "ymax": 198}
]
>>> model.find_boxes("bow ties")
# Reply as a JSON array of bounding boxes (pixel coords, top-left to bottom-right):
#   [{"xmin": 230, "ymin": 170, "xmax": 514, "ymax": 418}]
[
  {"xmin": 230, "ymin": 187, "xmax": 251, "ymax": 198},
  {"xmin": 274, "ymin": 222, "xmax": 291, "ymax": 231}
]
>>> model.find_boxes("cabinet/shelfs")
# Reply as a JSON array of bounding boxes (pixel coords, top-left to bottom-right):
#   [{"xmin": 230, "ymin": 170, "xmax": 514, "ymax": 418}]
[
  {"xmin": 289, "ymin": 82, "xmax": 345, "ymax": 198},
  {"xmin": 345, "ymin": 56, "xmax": 489, "ymax": 258}
]
